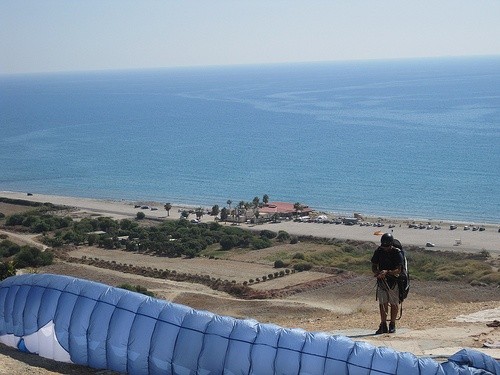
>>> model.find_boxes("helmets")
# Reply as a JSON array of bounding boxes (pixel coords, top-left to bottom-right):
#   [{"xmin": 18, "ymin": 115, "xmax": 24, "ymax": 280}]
[{"xmin": 381, "ymin": 233, "xmax": 394, "ymax": 243}]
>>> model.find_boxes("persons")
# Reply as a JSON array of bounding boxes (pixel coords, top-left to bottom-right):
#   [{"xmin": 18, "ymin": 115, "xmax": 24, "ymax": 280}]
[{"xmin": 370, "ymin": 234, "xmax": 404, "ymax": 334}]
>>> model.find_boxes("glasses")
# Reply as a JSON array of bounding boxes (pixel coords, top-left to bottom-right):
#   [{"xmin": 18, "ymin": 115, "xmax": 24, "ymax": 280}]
[{"xmin": 381, "ymin": 244, "xmax": 391, "ymax": 247}]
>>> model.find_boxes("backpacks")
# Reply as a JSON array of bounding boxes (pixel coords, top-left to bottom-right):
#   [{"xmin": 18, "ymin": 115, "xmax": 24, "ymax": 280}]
[{"xmin": 392, "ymin": 239, "xmax": 410, "ymax": 304}]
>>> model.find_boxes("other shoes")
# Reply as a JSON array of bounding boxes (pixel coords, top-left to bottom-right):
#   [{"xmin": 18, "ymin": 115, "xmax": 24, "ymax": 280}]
[
  {"xmin": 389, "ymin": 322, "xmax": 395, "ymax": 333},
  {"xmin": 376, "ymin": 323, "xmax": 388, "ymax": 335}
]
